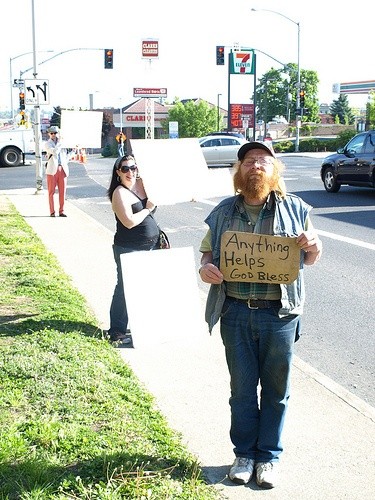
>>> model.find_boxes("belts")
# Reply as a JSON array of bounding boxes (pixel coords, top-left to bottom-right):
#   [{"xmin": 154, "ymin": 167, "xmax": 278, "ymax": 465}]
[{"xmin": 225, "ymin": 295, "xmax": 282, "ymax": 309}]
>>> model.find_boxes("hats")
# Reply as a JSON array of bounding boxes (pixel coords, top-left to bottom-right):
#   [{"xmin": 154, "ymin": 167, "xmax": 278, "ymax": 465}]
[
  {"xmin": 47, "ymin": 126, "xmax": 61, "ymax": 133},
  {"xmin": 237, "ymin": 141, "xmax": 277, "ymax": 161}
]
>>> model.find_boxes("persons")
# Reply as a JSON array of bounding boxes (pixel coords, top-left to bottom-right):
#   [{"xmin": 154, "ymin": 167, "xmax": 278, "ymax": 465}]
[
  {"xmin": 44, "ymin": 126, "xmax": 70, "ymax": 217},
  {"xmin": 107, "ymin": 154, "xmax": 160, "ymax": 344},
  {"xmin": 118, "ymin": 134, "xmax": 124, "ymax": 156},
  {"xmin": 201, "ymin": 141, "xmax": 323, "ymax": 489}
]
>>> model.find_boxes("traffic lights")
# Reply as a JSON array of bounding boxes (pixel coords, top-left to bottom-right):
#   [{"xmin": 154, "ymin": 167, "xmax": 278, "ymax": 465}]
[
  {"xmin": 216, "ymin": 46, "xmax": 224, "ymax": 65},
  {"xmin": 105, "ymin": 48, "xmax": 113, "ymax": 69},
  {"xmin": 300, "ymin": 91, "xmax": 305, "ymax": 107},
  {"xmin": 20, "ymin": 93, "xmax": 25, "ymax": 110}
]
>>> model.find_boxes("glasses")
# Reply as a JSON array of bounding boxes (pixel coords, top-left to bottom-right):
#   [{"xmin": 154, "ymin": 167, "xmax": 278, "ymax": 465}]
[
  {"xmin": 242, "ymin": 156, "xmax": 272, "ymax": 164},
  {"xmin": 50, "ymin": 132, "xmax": 56, "ymax": 135},
  {"xmin": 119, "ymin": 165, "xmax": 137, "ymax": 174}
]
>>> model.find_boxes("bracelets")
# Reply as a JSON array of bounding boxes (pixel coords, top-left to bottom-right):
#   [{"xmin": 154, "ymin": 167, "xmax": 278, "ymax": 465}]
[{"xmin": 199, "ymin": 262, "xmax": 208, "ymax": 273}]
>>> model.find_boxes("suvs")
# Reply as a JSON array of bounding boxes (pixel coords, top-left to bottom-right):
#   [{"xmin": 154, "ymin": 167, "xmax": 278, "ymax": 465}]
[
  {"xmin": 319, "ymin": 131, "xmax": 375, "ymax": 193},
  {"xmin": 206, "ymin": 131, "xmax": 245, "ymax": 140}
]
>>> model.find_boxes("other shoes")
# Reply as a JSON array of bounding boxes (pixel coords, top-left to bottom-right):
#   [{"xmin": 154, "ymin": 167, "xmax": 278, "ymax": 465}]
[
  {"xmin": 106, "ymin": 330, "xmax": 132, "ymax": 344},
  {"xmin": 51, "ymin": 214, "xmax": 55, "ymax": 217},
  {"xmin": 59, "ymin": 214, "xmax": 67, "ymax": 217}
]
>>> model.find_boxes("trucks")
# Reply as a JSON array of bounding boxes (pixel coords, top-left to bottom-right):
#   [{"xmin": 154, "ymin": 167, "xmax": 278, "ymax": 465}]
[{"xmin": 0, "ymin": 129, "xmax": 35, "ymax": 167}]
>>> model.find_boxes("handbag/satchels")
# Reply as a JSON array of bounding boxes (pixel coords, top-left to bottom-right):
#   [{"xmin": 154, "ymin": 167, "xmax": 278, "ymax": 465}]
[
  {"xmin": 157, "ymin": 230, "xmax": 171, "ymax": 249},
  {"xmin": 45, "ymin": 162, "xmax": 48, "ymax": 168}
]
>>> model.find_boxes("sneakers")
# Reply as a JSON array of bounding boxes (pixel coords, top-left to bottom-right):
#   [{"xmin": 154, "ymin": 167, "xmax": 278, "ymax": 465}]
[
  {"xmin": 255, "ymin": 462, "xmax": 279, "ymax": 488},
  {"xmin": 229, "ymin": 456, "xmax": 255, "ymax": 485}
]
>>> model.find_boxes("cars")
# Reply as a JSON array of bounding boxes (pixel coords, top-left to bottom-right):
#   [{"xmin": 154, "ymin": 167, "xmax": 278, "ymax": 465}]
[{"xmin": 198, "ymin": 135, "xmax": 250, "ymax": 166}]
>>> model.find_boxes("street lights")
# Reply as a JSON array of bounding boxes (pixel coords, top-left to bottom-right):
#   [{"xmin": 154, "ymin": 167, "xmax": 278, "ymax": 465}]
[
  {"xmin": 10, "ymin": 51, "xmax": 53, "ymax": 118},
  {"xmin": 218, "ymin": 94, "xmax": 222, "ymax": 131},
  {"xmin": 252, "ymin": 8, "xmax": 301, "ymax": 154}
]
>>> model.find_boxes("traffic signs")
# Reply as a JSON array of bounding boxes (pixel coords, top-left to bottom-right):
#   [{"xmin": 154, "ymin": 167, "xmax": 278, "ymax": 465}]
[
  {"xmin": 24, "ymin": 79, "xmax": 49, "ymax": 105},
  {"xmin": 13, "ymin": 79, "xmax": 24, "ymax": 84}
]
